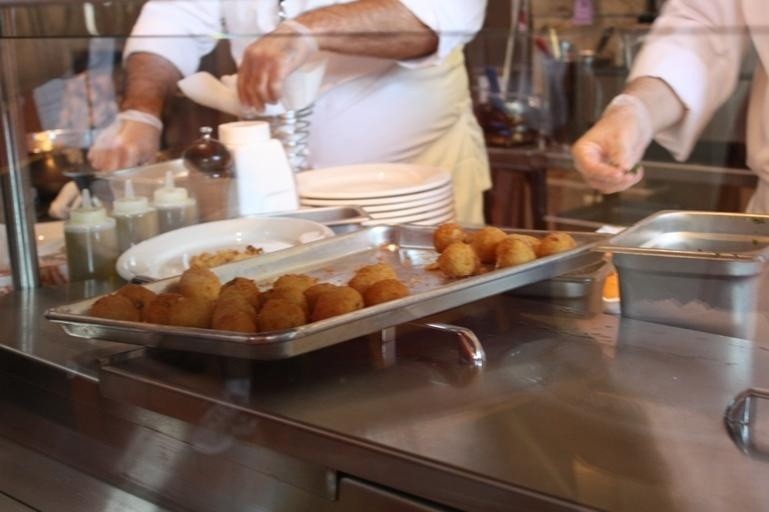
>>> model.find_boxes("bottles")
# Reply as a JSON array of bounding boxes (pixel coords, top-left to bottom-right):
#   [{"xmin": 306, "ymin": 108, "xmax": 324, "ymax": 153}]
[
  {"xmin": 544, "ymin": 45, "xmax": 612, "ymax": 147},
  {"xmin": 63, "ymin": 120, "xmax": 270, "ymax": 283}
]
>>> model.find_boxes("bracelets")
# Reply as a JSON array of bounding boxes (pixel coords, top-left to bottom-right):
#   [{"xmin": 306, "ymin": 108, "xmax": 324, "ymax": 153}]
[
  {"xmin": 274, "ymin": 19, "xmax": 320, "ymax": 58},
  {"xmin": 110, "ymin": 105, "xmax": 165, "ymax": 140},
  {"xmin": 602, "ymin": 88, "xmax": 656, "ymax": 147}
]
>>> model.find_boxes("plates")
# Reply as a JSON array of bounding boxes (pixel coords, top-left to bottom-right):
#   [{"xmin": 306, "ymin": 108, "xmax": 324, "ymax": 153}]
[
  {"xmin": 293, "ymin": 163, "xmax": 456, "ymax": 227},
  {"xmin": 32, "ymin": 221, "xmax": 66, "ymax": 258},
  {"xmin": 115, "ymin": 217, "xmax": 335, "ymax": 286}
]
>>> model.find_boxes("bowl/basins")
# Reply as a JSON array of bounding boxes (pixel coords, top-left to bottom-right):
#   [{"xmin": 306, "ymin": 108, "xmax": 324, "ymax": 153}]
[
  {"xmin": 223, "ymin": 58, "xmax": 328, "ymax": 111},
  {"xmin": 474, "ymin": 91, "xmax": 545, "ymax": 148}
]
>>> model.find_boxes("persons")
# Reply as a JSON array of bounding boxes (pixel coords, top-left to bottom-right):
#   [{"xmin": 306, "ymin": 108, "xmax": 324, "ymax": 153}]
[
  {"xmin": 85, "ymin": 2, "xmax": 494, "ymax": 226},
  {"xmin": 568, "ymin": 0, "xmax": 769, "ymax": 218}
]
